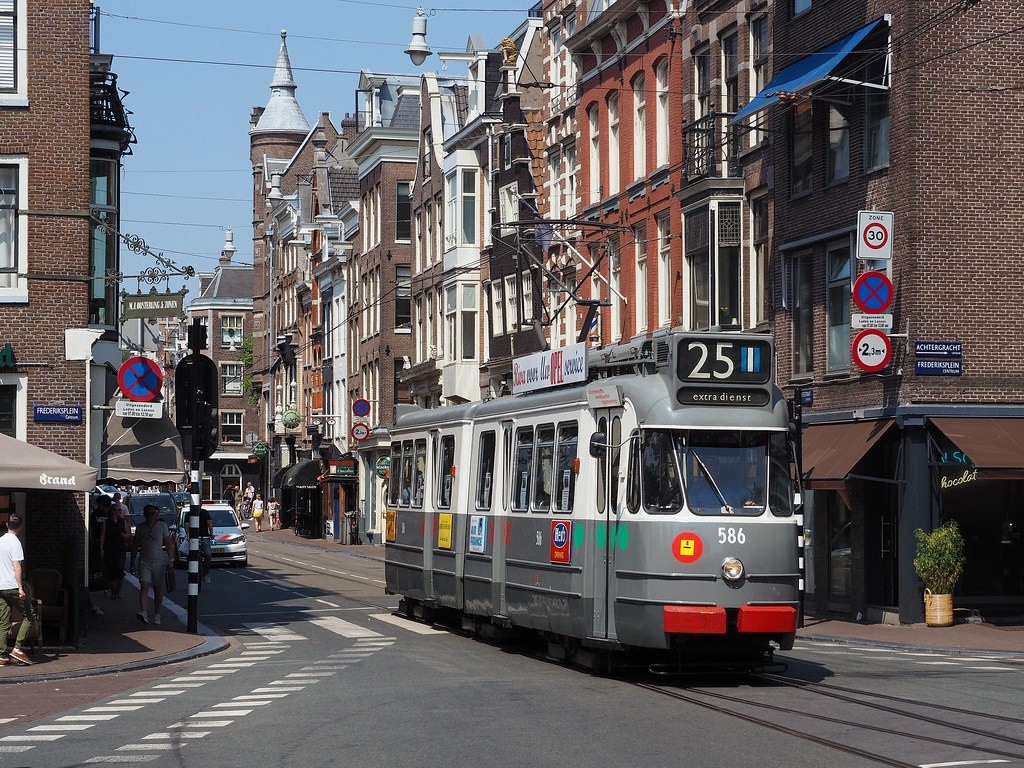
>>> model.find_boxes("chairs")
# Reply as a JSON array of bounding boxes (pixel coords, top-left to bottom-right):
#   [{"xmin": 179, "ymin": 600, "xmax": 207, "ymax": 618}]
[
  {"xmin": 24, "ymin": 568, "xmax": 68, "ymax": 643},
  {"xmin": 4, "ymin": 579, "xmax": 44, "ymax": 648}
]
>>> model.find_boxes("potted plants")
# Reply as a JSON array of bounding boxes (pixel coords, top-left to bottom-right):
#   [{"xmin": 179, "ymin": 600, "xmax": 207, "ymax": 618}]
[{"xmin": 912, "ymin": 518, "xmax": 967, "ymax": 627}]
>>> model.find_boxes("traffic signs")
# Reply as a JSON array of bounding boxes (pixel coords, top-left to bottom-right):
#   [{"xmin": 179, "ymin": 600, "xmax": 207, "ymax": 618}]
[{"xmin": 916, "ymin": 342, "xmax": 963, "ymax": 357}]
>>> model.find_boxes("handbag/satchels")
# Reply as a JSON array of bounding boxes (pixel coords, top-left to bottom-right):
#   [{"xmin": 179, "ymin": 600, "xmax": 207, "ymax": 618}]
[{"xmin": 165, "ymin": 568, "xmax": 177, "ymax": 593}]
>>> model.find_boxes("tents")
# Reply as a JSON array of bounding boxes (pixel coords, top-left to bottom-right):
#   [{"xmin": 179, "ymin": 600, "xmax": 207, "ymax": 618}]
[{"xmin": 0, "ymin": 432, "xmax": 104, "ymax": 616}]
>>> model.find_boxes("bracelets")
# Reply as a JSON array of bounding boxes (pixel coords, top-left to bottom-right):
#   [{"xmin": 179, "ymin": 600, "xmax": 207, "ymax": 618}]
[{"xmin": 168, "ymin": 558, "xmax": 174, "ymax": 561}]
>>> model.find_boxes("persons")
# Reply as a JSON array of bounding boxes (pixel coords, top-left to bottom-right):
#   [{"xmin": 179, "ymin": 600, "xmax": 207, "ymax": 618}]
[
  {"xmin": 222, "ymin": 485, "xmax": 234, "ymax": 506},
  {"xmin": 184, "ymin": 497, "xmax": 213, "ymax": 584},
  {"xmin": 242, "ymin": 482, "xmax": 255, "ymax": 514},
  {"xmin": 267, "ymin": 496, "xmax": 280, "ymax": 531},
  {"xmin": 129, "ymin": 505, "xmax": 175, "ymax": 625},
  {"xmin": 234, "ymin": 486, "xmax": 244, "ymax": 521},
  {"xmin": 89, "ymin": 492, "xmax": 132, "ymax": 600},
  {"xmin": 0, "ymin": 514, "xmax": 36, "ymax": 667},
  {"xmin": 251, "ymin": 494, "xmax": 263, "ymax": 533},
  {"xmin": 687, "ymin": 453, "xmax": 757, "ymax": 507}
]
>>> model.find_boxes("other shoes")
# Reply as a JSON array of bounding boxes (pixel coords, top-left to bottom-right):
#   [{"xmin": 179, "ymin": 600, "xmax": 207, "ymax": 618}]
[
  {"xmin": 259, "ymin": 529, "xmax": 261, "ymax": 532},
  {"xmin": 201, "ymin": 575, "xmax": 211, "ymax": 583},
  {"xmin": 91, "ymin": 606, "xmax": 105, "ymax": 615},
  {"xmin": 256, "ymin": 530, "xmax": 258, "ymax": 533},
  {"xmin": 0, "ymin": 657, "xmax": 19, "ymax": 665},
  {"xmin": 109, "ymin": 592, "xmax": 118, "ymax": 600},
  {"xmin": 9, "ymin": 650, "xmax": 33, "ymax": 665},
  {"xmin": 152, "ymin": 614, "xmax": 162, "ymax": 624},
  {"xmin": 103, "ymin": 589, "xmax": 112, "ymax": 599},
  {"xmin": 135, "ymin": 609, "xmax": 149, "ymax": 624},
  {"xmin": 272, "ymin": 527, "xmax": 274, "ymax": 531}
]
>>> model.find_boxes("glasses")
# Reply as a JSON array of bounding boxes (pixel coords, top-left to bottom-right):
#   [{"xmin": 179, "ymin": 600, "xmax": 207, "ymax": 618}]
[{"xmin": 113, "ymin": 508, "xmax": 123, "ymax": 512}]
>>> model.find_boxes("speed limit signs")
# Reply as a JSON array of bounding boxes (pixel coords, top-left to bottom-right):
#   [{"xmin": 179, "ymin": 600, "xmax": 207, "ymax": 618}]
[{"xmin": 855, "ymin": 211, "xmax": 893, "ymax": 261}]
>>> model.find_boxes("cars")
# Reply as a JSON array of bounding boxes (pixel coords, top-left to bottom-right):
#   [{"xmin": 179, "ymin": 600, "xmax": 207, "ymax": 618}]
[
  {"xmin": 173, "ymin": 502, "xmax": 250, "ymax": 567},
  {"xmin": 123, "ymin": 490, "xmax": 179, "ymax": 550}
]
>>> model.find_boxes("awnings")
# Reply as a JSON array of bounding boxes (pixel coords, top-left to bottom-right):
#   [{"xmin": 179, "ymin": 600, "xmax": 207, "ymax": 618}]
[
  {"xmin": 100, "ymin": 372, "xmax": 185, "ymax": 485},
  {"xmin": 280, "ymin": 463, "xmax": 296, "ymax": 489},
  {"xmin": 791, "ymin": 417, "xmax": 908, "ymax": 492},
  {"xmin": 284, "ymin": 460, "xmax": 321, "ymax": 489},
  {"xmin": 925, "ymin": 417, "xmax": 1024, "ymax": 480},
  {"xmin": 271, "ymin": 467, "xmax": 289, "ymax": 488},
  {"xmin": 725, "ymin": 20, "xmax": 892, "ymax": 136}
]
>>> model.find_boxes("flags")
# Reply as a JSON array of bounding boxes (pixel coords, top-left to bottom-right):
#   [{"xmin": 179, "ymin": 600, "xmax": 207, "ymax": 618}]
[{"xmin": 532, "ymin": 219, "xmax": 554, "ymax": 251}]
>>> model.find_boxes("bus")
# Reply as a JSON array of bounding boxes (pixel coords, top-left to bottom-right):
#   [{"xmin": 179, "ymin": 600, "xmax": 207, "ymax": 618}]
[{"xmin": 381, "ymin": 323, "xmax": 805, "ymax": 681}]
